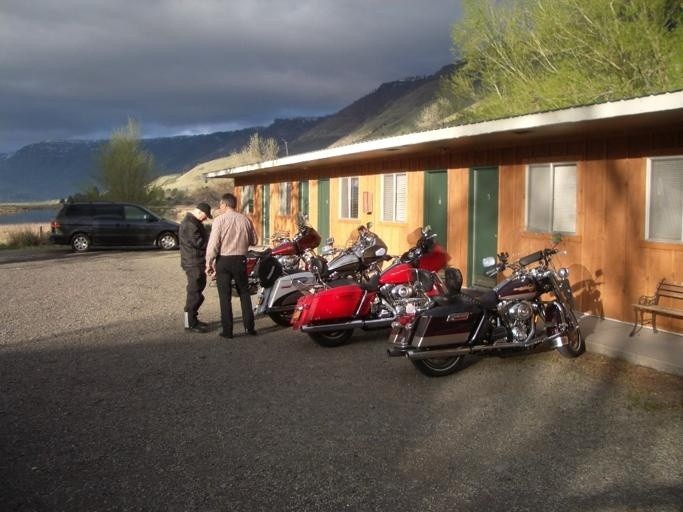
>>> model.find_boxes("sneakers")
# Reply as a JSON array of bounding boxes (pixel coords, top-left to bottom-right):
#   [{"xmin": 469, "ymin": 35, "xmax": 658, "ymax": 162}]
[
  {"xmin": 219, "ymin": 329, "xmax": 258, "ymax": 339},
  {"xmin": 185, "ymin": 321, "xmax": 208, "ymax": 333}
]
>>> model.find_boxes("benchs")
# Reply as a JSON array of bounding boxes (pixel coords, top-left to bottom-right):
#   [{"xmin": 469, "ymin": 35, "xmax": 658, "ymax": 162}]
[{"xmin": 628, "ymin": 277, "xmax": 682, "ymax": 337}]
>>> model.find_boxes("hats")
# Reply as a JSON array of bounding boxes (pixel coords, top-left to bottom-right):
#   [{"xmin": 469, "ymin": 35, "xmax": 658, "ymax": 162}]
[{"xmin": 196, "ymin": 203, "xmax": 213, "ymax": 219}]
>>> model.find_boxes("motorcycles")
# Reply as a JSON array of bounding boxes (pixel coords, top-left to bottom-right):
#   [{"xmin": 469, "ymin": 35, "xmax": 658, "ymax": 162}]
[
  {"xmin": 386, "ymin": 231, "xmax": 585, "ymax": 377},
  {"xmin": 253, "ymin": 222, "xmax": 387, "ymax": 328},
  {"xmin": 209, "ymin": 224, "xmax": 321, "ymax": 296},
  {"xmin": 290, "ymin": 225, "xmax": 448, "ymax": 346}
]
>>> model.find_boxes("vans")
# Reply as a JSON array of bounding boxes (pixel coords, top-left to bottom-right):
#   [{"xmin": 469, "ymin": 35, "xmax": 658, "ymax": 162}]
[{"xmin": 50, "ymin": 199, "xmax": 182, "ymax": 252}]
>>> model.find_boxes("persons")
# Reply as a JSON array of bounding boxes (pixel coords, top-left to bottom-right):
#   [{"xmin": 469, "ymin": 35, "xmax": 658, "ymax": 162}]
[
  {"xmin": 204, "ymin": 192, "xmax": 257, "ymax": 338},
  {"xmin": 178, "ymin": 203, "xmax": 213, "ymax": 333}
]
